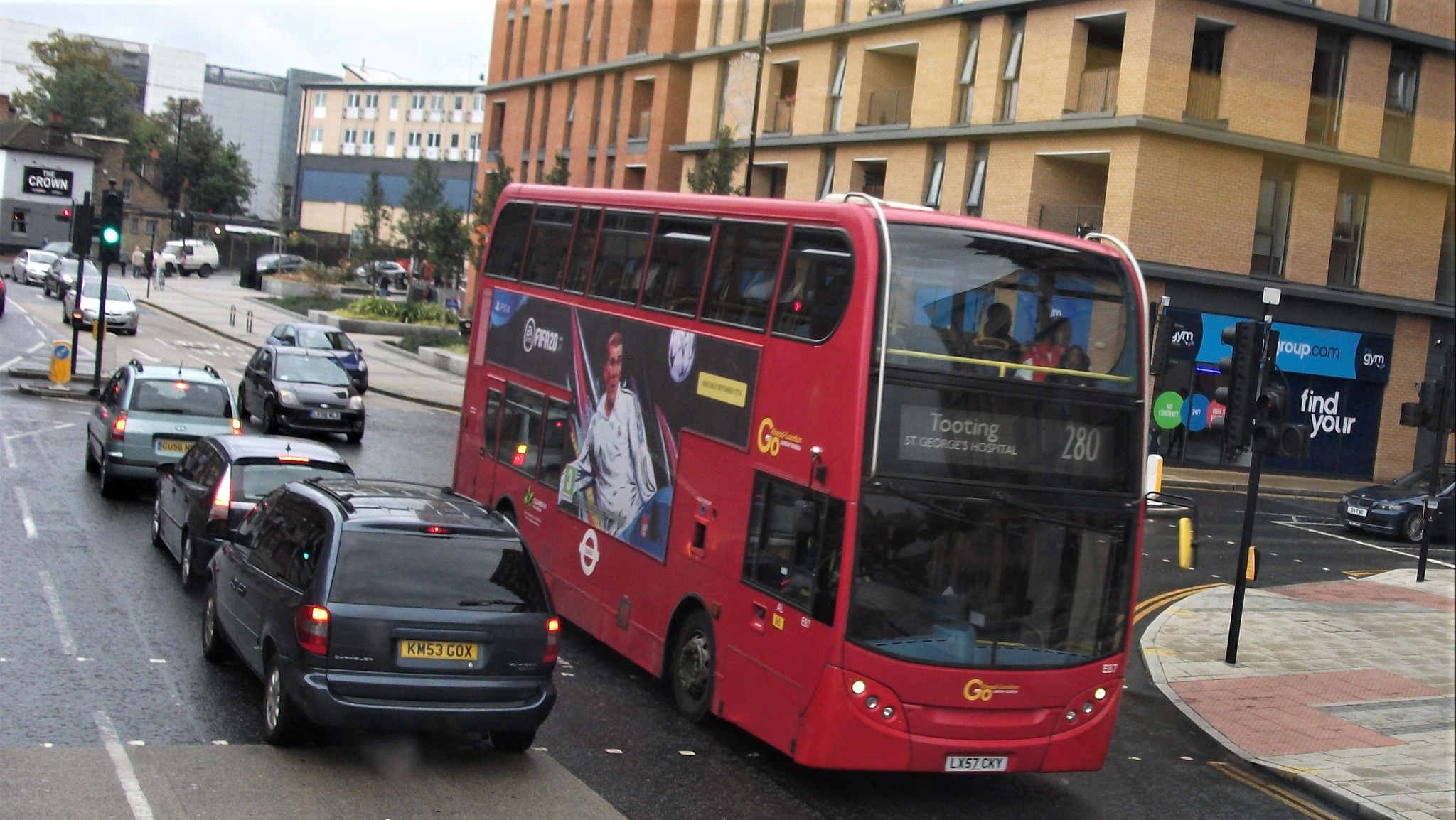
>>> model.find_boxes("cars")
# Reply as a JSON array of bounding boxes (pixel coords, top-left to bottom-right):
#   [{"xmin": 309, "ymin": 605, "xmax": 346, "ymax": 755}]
[
  {"xmin": 489, "ymin": 405, "xmax": 569, "ymax": 489},
  {"xmin": 12, "ymin": 248, "xmax": 60, "ymax": 286},
  {"xmin": 43, "ymin": 241, "xmax": 80, "ymax": 260},
  {"xmin": 256, "ymin": 253, "xmax": 306, "ymax": 278},
  {"xmin": 62, "ymin": 279, "xmax": 138, "ymax": 336},
  {"xmin": 151, "ymin": 433, "xmax": 359, "ymax": 597},
  {"xmin": 1337, "ymin": 463, "xmax": 1456, "ymax": 544},
  {"xmin": 0, "ymin": 269, "xmax": 11, "ymax": 317},
  {"xmin": 357, "ymin": 260, "xmax": 406, "ymax": 278},
  {"xmin": 43, "ymin": 257, "xmax": 101, "ymax": 301},
  {"xmin": 237, "ymin": 345, "xmax": 365, "ymax": 445},
  {"xmin": 84, "ymin": 358, "xmax": 243, "ymax": 500},
  {"xmin": 265, "ymin": 322, "xmax": 369, "ymax": 396}
]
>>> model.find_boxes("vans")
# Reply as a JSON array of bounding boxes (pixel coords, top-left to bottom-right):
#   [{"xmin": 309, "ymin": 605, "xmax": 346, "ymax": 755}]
[{"xmin": 161, "ymin": 239, "xmax": 221, "ymax": 279}]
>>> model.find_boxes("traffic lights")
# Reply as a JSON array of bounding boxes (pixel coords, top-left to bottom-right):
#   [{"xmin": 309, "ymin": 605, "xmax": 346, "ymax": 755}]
[
  {"xmin": 98, "ymin": 189, "xmax": 125, "ymax": 263},
  {"xmin": 1251, "ymin": 387, "xmax": 1311, "ymax": 461},
  {"xmin": 1211, "ymin": 320, "xmax": 1266, "ymax": 448},
  {"xmin": 1150, "ymin": 318, "xmax": 1184, "ymax": 374}
]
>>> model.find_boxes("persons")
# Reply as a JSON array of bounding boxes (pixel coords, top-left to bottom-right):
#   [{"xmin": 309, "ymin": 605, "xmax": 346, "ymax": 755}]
[
  {"xmin": 377, "ymin": 270, "xmax": 392, "ymax": 298},
  {"xmin": 119, "ymin": 244, "xmax": 186, "ymax": 286},
  {"xmin": 557, "ymin": 331, "xmax": 673, "ymax": 559},
  {"xmin": 971, "ymin": 302, "xmax": 1097, "ymax": 386}
]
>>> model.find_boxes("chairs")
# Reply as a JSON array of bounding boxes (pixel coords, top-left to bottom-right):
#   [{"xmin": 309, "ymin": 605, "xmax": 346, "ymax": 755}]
[{"xmin": 493, "ymin": 203, "xmax": 855, "ymax": 340}]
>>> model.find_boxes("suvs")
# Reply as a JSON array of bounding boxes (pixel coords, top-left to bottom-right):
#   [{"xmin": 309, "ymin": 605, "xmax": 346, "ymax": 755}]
[{"xmin": 202, "ymin": 477, "xmax": 561, "ymax": 756}]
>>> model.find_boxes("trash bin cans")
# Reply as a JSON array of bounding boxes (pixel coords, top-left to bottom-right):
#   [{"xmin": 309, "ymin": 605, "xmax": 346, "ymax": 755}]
[
  {"xmin": 395, "ymin": 272, "xmax": 409, "ymax": 290},
  {"xmin": 458, "ymin": 320, "xmax": 472, "ymax": 336},
  {"xmin": 240, "ymin": 259, "xmax": 258, "ymax": 287}
]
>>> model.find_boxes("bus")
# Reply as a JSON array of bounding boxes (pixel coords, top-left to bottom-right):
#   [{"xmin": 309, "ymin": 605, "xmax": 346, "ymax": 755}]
[{"xmin": 452, "ymin": 192, "xmax": 1203, "ymax": 772}]
